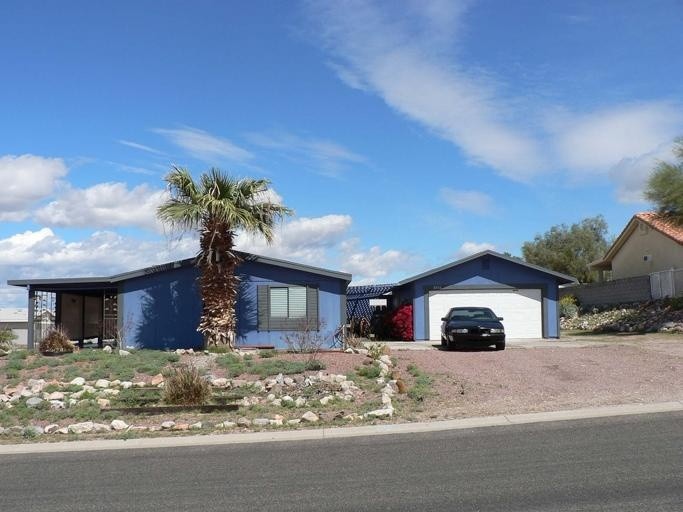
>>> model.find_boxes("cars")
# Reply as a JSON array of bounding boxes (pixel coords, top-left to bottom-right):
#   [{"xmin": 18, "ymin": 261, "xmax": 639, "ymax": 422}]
[{"xmin": 441, "ymin": 307, "xmax": 505, "ymax": 350}]
